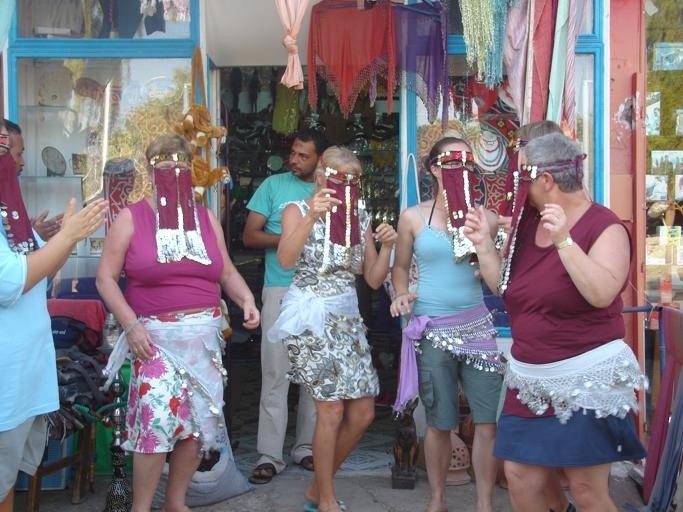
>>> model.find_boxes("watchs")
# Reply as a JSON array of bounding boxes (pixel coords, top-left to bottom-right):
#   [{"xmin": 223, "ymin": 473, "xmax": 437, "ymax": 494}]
[{"xmin": 555, "ymin": 237, "xmax": 574, "ymax": 249}]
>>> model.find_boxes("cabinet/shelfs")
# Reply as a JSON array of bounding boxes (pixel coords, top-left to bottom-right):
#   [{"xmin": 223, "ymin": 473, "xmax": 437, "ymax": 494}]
[{"xmin": 19, "ymin": 101, "xmax": 107, "ymax": 261}]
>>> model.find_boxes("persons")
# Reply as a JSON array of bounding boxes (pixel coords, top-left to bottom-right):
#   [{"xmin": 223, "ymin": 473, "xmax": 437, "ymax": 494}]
[
  {"xmin": 465, "ymin": 135, "xmax": 647, "ymax": 512},
  {"xmin": 474, "ymin": 120, "xmax": 575, "ymax": 512},
  {"xmin": 0, "ymin": 119, "xmax": 77, "ymax": 251},
  {"xmin": 0, "ymin": 116, "xmax": 112, "ymax": 509},
  {"xmin": 240, "ymin": 129, "xmax": 330, "ymax": 482},
  {"xmin": 275, "ymin": 145, "xmax": 396, "ymax": 512},
  {"xmin": 392, "ymin": 138, "xmax": 505, "ymax": 512},
  {"xmin": 93, "ymin": 134, "xmax": 262, "ymax": 510}
]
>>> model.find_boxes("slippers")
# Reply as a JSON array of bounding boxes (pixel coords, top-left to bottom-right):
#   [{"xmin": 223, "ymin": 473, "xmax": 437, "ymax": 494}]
[
  {"xmin": 248, "ymin": 463, "xmax": 276, "ymax": 485},
  {"xmin": 304, "ymin": 499, "xmax": 348, "ymax": 512},
  {"xmin": 299, "ymin": 454, "xmax": 316, "ymax": 471}
]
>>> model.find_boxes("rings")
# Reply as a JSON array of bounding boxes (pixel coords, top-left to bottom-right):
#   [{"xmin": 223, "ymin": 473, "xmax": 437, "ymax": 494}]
[{"xmin": 134, "ymin": 346, "xmax": 140, "ymax": 353}]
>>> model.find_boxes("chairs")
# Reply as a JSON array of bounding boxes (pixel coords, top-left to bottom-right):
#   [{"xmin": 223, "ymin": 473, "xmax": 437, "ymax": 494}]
[{"xmin": 15, "ymin": 296, "xmax": 115, "ymax": 511}]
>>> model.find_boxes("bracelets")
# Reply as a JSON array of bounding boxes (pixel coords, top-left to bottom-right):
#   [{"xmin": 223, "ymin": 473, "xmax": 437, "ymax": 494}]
[{"xmin": 125, "ymin": 319, "xmax": 142, "ymax": 336}]
